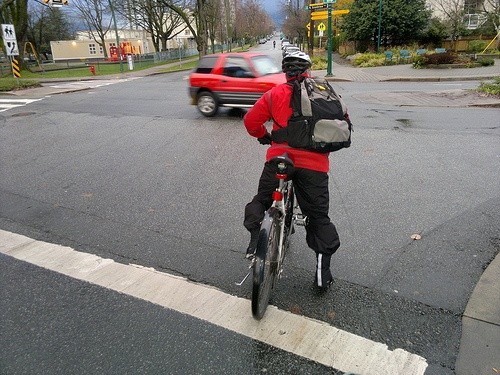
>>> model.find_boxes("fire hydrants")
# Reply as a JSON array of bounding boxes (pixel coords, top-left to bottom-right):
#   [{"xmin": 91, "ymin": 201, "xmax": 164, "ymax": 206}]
[{"xmin": 90, "ymin": 64, "xmax": 96, "ymax": 76}]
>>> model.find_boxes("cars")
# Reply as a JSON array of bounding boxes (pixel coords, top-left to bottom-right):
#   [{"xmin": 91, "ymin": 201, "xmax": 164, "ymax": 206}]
[{"xmin": 259, "ymin": 33, "xmax": 301, "ymax": 58}]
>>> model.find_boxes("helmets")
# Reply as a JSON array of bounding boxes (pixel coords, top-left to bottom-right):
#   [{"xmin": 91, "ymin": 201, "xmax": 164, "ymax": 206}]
[{"xmin": 282, "ymin": 51, "xmax": 312, "ymax": 68}]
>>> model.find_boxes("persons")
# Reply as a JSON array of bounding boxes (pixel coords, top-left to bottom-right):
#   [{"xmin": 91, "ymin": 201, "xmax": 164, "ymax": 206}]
[
  {"xmin": 281, "ymin": 38, "xmax": 283, "ymax": 50},
  {"xmin": 273, "ymin": 41, "xmax": 276, "ymax": 48},
  {"xmin": 242, "ymin": 51, "xmax": 341, "ymax": 287}
]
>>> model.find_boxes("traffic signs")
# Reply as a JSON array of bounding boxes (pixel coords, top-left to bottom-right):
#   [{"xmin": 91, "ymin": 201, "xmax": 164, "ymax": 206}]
[{"xmin": 304, "ymin": 0, "xmax": 351, "ymax": 21}]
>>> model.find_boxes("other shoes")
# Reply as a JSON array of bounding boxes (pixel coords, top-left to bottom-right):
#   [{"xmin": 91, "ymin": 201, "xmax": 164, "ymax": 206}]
[
  {"xmin": 314, "ymin": 253, "xmax": 332, "ymax": 287},
  {"xmin": 246, "ymin": 241, "xmax": 257, "ymax": 259}
]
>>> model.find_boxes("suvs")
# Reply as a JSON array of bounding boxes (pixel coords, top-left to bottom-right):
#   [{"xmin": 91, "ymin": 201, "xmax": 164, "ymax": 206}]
[{"xmin": 182, "ymin": 50, "xmax": 319, "ymax": 117}]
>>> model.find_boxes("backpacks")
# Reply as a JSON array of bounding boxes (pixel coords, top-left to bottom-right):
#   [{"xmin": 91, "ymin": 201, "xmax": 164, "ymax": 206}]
[{"xmin": 270, "ymin": 78, "xmax": 351, "ymax": 153}]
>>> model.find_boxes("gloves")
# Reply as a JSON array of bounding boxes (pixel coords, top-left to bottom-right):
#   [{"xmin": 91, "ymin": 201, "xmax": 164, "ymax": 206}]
[{"xmin": 258, "ymin": 130, "xmax": 271, "ymax": 144}]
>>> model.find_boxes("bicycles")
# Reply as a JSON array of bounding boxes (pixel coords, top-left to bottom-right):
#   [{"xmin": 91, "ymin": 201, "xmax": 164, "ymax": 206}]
[{"xmin": 233, "ymin": 135, "xmax": 310, "ymax": 322}]
